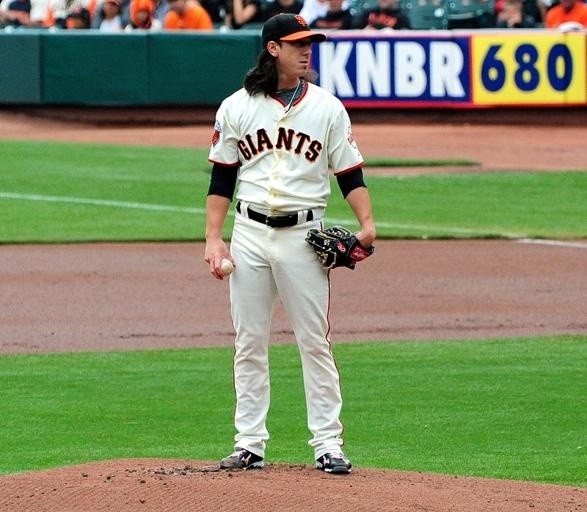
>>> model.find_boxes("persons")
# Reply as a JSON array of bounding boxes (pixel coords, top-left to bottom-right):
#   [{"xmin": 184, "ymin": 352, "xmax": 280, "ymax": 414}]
[
  {"xmin": 0, "ymin": 0, "xmax": 587, "ymax": 29},
  {"xmin": 202, "ymin": 14, "xmax": 376, "ymax": 475}
]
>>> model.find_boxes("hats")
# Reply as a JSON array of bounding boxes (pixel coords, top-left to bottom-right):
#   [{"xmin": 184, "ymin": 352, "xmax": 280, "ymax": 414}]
[{"xmin": 262, "ymin": 13, "xmax": 327, "ymax": 48}]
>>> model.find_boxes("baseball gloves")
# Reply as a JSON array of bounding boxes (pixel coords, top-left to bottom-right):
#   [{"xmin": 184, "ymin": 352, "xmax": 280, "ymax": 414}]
[{"xmin": 305, "ymin": 222, "xmax": 374, "ymax": 270}]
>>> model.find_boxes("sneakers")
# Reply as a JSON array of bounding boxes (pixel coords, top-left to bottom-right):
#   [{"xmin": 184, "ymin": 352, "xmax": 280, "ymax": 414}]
[
  {"xmin": 220, "ymin": 448, "xmax": 265, "ymax": 469},
  {"xmin": 316, "ymin": 452, "xmax": 352, "ymax": 473}
]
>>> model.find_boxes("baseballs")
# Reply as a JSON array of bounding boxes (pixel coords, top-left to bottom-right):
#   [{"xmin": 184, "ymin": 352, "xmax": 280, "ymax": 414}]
[{"xmin": 221, "ymin": 259, "xmax": 232, "ymax": 276}]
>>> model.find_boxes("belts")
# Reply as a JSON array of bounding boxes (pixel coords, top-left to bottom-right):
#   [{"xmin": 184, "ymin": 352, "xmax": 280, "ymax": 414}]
[{"xmin": 236, "ymin": 201, "xmax": 313, "ymax": 228}]
